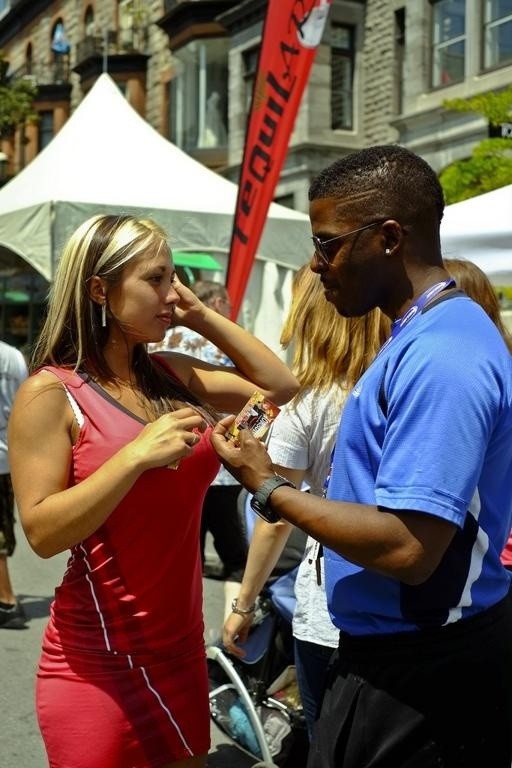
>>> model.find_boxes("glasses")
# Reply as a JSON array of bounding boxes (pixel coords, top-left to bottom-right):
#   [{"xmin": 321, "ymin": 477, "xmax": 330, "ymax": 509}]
[{"xmin": 312, "ymin": 222, "xmax": 409, "ymax": 264}]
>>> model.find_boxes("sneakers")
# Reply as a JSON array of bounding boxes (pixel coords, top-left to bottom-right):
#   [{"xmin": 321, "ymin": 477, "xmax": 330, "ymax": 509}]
[{"xmin": 0, "ymin": 598, "xmax": 27, "ymax": 629}]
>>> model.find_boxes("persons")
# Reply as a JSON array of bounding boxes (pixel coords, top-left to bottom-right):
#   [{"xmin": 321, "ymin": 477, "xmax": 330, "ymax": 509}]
[
  {"xmin": 10, "ymin": 213, "xmax": 298, "ymax": 768},
  {"xmin": 214, "ymin": 148, "xmax": 511, "ymax": 768},
  {"xmin": 141, "ymin": 283, "xmax": 251, "ymax": 578},
  {"xmin": 1, "ymin": 312, "xmax": 29, "ymax": 629}
]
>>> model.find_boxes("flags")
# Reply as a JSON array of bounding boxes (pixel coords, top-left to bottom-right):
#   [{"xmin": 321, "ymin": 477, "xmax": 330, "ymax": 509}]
[{"xmin": 228, "ymin": 1, "xmax": 331, "ymax": 324}]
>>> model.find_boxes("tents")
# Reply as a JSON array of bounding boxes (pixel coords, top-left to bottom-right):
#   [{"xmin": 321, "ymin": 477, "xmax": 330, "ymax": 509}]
[{"xmin": 5, "ymin": 72, "xmax": 314, "ymax": 371}]
